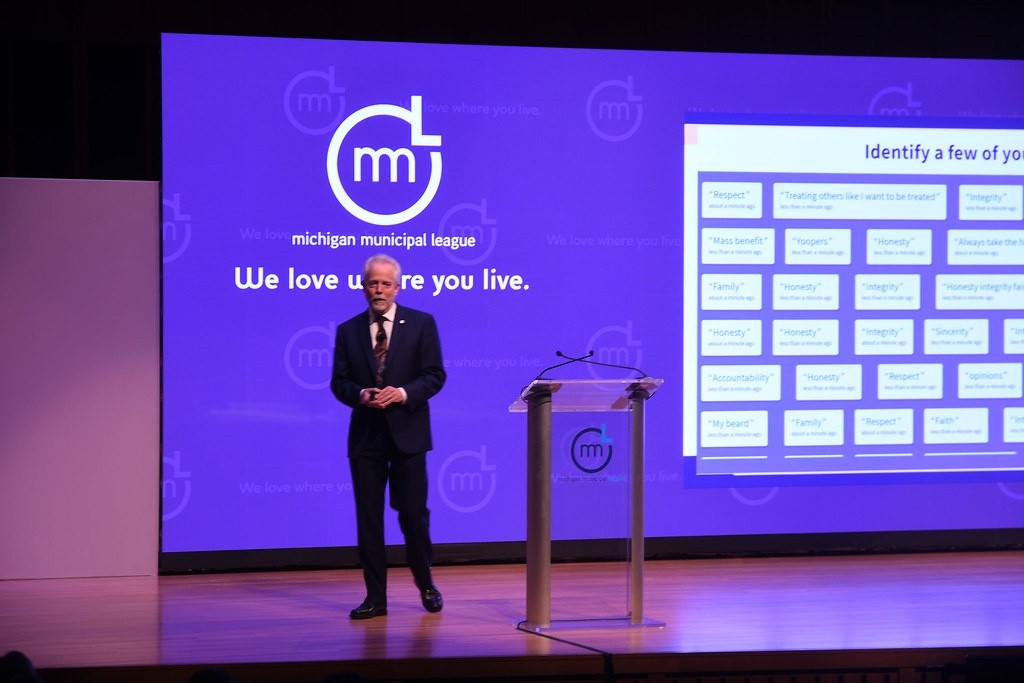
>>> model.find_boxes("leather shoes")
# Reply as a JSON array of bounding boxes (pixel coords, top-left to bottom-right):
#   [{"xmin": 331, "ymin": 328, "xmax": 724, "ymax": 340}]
[
  {"xmin": 350, "ymin": 601, "xmax": 388, "ymax": 619},
  {"xmin": 414, "ymin": 578, "xmax": 443, "ymax": 612}
]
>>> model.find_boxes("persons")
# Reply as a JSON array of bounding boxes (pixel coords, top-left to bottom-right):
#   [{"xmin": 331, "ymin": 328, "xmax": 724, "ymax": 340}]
[{"xmin": 330, "ymin": 253, "xmax": 446, "ymax": 620}]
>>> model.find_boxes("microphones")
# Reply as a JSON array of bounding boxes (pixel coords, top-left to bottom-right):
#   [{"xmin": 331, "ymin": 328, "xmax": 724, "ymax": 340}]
[
  {"xmin": 536, "ymin": 350, "xmax": 594, "ymax": 379},
  {"xmin": 556, "ymin": 350, "xmax": 648, "ymax": 377}
]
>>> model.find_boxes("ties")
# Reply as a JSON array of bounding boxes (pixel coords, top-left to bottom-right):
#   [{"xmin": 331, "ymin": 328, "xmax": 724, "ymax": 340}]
[{"xmin": 374, "ymin": 316, "xmax": 390, "ymax": 389}]
